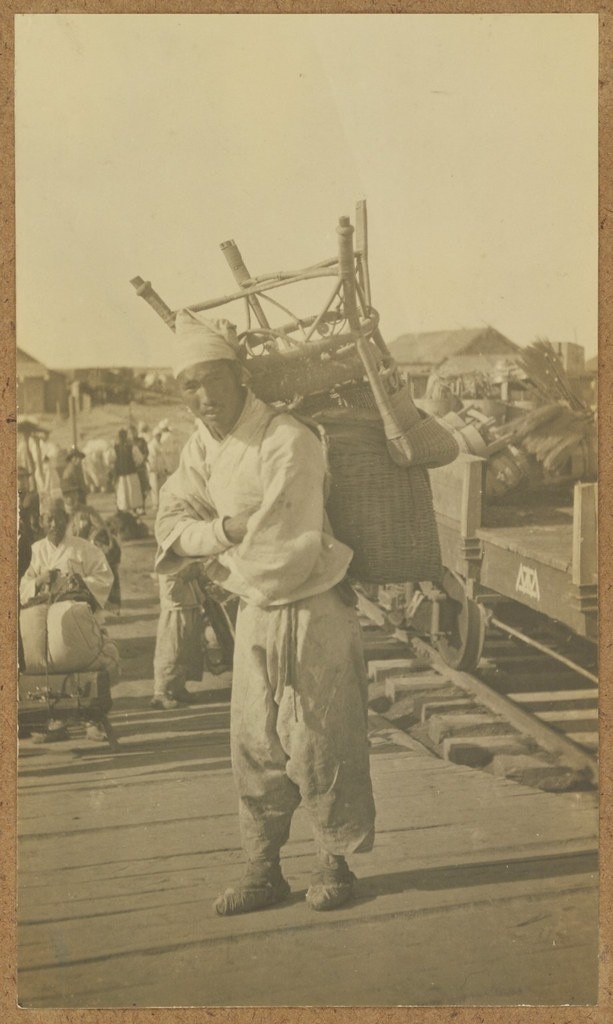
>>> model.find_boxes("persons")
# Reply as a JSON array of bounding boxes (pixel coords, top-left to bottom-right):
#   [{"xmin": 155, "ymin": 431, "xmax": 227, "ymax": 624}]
[
  {"xmin": 153, "ymin": 308, "xmax": 377, "ymax": 916},
  {"xmin": 114, "ymin": 425, "xmax": 151, "ymax": 518},
  {"xmin": 139, "ymin": 418, "xmax": 181, "ymax": 508},
  {"xmin": 149, "ymin": 544, "xmax": 212, "ymax": 709},
  {"xmin": 16, "ymin": 449, "xmax": 119, "ymax": 745}
]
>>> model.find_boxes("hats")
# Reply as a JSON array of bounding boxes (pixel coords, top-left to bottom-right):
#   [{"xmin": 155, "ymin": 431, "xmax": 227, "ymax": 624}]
[
  {"xmin": 118, "ymin": 428, "xmax": 127, "ymax": 435},
  {"xmin": 138, "ymin": 421, "xmax": 147, "ymax": 430},
  {"xmin": 172, "ymin": 308, "xmax": 239, "ymax": 378},
  {"xmin": 66, "ymin": 449, "xmax": 85, "ymax": 461},
  {"xmin": 40, "ymin": 497, "xmax": 65, "ymax": 515},
  {"xmin": 159, "ymin": 418, "xmax": 169, "ymax": 429},
  {"xmin": 152, "ymin": 427, "xmax": 161, "ymax": 436}
]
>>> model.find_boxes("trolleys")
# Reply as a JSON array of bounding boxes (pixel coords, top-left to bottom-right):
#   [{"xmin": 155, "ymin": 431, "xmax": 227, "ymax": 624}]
[{"xmin": 17, "ymin": 567, "xmax": 118, "ymax": 753}]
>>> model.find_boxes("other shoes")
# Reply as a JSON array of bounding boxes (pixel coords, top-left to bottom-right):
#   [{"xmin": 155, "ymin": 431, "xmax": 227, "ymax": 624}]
[
  {"xmin": 214, "ymin": 876, "xmax": 290, "ymax": 915},
  {"xmin": 305, "ymin": 870, "xmax": 356, "ymax": 910},
  {"xmin": 171, "ymin": 689, "xmax": 198, "ymax": 702},
  {"xmin": 33, "ymin": 726, "xmax": 68, "ymax": 743},
  {"xmin": 150, "ymin": 689, "xmax": 180, "ymax": 708},
  {"xmin": 86, "ymin": 722, "xmax": 108, "ymax": 741}
]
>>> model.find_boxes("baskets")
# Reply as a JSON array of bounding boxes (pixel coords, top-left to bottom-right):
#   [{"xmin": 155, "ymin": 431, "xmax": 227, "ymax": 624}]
[{"xmin": 279, "ymin": 386, "xmax": 443, "ymax": 585}]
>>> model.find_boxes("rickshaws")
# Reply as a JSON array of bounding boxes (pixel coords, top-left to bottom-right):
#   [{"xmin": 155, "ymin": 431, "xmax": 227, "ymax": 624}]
[{"xmin": 407, "ymin": 389, "xmax": 600, "ymax": 670}]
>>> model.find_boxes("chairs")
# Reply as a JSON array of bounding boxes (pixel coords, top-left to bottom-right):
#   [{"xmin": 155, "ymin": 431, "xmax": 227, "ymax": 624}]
[{"xmin": 125, "ymin": 198, "xmax": 463, "ymax": 469}]
